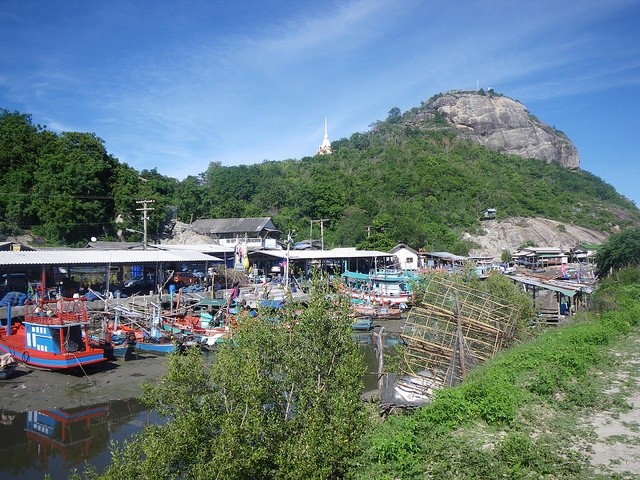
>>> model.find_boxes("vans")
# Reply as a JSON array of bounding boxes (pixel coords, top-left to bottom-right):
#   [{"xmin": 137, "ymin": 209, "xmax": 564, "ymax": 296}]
[{"xmin": 172, "ymin": 271, "xmax": 198, "ymax": 288}]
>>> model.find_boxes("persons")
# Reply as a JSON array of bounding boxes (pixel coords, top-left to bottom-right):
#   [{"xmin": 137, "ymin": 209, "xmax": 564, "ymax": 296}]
[{"xmin": 123, "ymin": 332, "xmax": 139, "ymax": 360}]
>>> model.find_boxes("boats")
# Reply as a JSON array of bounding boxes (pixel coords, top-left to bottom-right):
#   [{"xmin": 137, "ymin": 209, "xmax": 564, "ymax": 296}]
[
  {"xmin": 251, "ymin": 267, "xmax": 422, "ymax": 331},
  {"xmin": 24, "ymin": 402, "xmax": 109, "ymax": 470},
  {"xmin": 0, "ymin": 269, "xmax": 109, "ymax": 369},
  {"xmin": 84, "ymin": 292, "xmax": 247, "ymax": 357},
  {"xmin": 424, "ymin": 243, "xmax": 595, "ymax": 325}
]
